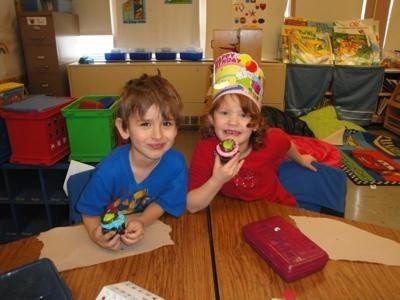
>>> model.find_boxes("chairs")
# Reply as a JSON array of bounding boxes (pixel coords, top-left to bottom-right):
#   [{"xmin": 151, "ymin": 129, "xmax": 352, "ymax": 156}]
[
  {"xmin": 66, "ymin": 167, "xmax": 99, "ymax": 221},
  {"xmin": 279, "ymin": 159, "xmax": 347, "ymax": 219}
]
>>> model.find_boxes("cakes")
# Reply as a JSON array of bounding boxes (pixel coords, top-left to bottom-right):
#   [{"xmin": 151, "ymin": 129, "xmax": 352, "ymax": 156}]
[
  {"xmin": 100, "ymin": 212, "xmax": 125, "ymax": 235},
  {"xmin": 215, "ymin": 139, "xmax": 239, "ymax": 163}
]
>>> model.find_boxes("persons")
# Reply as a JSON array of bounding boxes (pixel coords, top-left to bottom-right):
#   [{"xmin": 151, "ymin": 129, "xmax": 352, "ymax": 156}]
[
  {"xmin": 187, "ymin": 52, "xmax": 317, "ymax": 217},
  {"xmin": 75, "ymin": 74, "xmax": 187, "ymax": 251}
]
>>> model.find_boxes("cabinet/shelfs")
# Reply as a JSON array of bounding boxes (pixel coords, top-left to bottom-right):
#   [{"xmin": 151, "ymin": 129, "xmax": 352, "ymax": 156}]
[
  {"xmin": 260, "ymin": 62, "xmax": 286, "ymax": 110},
  {"xmin": 1, "ymin": 164, "xmax": 72, "ymax": 239},
  {"xmin": 16, "ymin": 12, "xmax": 81, "ymax": 97},
  {"xmin": 288, "ymin": 62, "xmax": 400, "ymax": 125},
  {"xmin": 68, "ymin": 61, "xmax": 211, "ymax": 130}
]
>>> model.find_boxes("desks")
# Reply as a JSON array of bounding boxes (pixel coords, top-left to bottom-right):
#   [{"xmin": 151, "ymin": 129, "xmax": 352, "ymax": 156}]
[{"xmin": 1, "ymin": 195, "xmax": 399, "ymax": 300}]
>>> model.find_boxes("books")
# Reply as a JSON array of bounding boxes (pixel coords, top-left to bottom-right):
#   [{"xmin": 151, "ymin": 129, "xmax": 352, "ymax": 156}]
[{"xmin": 281, "ymin": 18, "xmax": 380, "ymax": 66}]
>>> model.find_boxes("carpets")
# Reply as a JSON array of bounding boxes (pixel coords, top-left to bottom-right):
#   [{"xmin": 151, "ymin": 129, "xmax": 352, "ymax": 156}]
[{"xmin": 331, "ymin": 128, "xmax": 400, "ymax": 188}]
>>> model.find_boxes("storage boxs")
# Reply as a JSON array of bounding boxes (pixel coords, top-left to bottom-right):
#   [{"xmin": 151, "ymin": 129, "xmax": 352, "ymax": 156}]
[
  {"xmin": 179, "ymin": 48, "xmax": 203, "ymax": 61},
  {"xmin": 129, "ymin": 48, "xmax": 153, "ymax": 60},
  {"xmin": 155, "ymin": 48, "xmax": 177, "ymax": 60},
  {"xmin": 105, "ymin": 48, "xmax": 127, "ymax": 60}
]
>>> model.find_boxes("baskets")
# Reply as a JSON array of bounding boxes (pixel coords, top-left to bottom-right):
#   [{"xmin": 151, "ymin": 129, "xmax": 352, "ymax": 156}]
[
  {"xmin": 63, "ymin": 93, "xmax": 122, "ymax": 163},
  {"xmin": 3, "ymin": 96, "xmax": 77, "ymax": 166}
]
